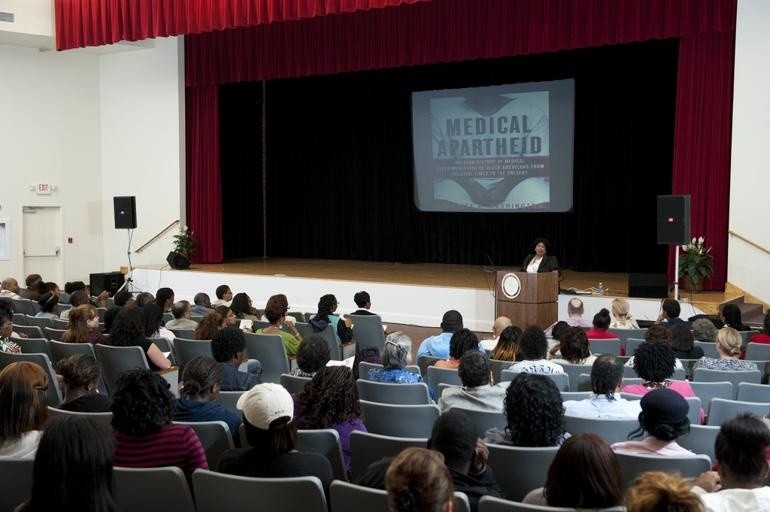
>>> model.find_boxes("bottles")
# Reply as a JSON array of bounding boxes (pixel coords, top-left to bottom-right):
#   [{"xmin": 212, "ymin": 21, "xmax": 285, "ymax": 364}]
[{"xmin": 598, "ymin": 282, "xmax": 603, "ymax": 294}]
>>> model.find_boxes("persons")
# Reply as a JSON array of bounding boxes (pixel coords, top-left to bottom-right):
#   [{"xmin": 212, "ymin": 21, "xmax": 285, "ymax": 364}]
[
  {"xmin": 483, "ymin": 297, "xmax": 770, "ymax": 511},
  {"xmin": 15, "ymin": 414, "xmax": 118, "ymax": 511},
  {"xmin": 309, "ymin": 294, "xmax": 352, "ymax": 343},
  {"xmin": 357, "ymin": 409, "xmax": 502, "ymax": 511},
  {"xmin": 435, "ymin": 328, "xmax": 478, "ymax": 369},
  {"xmin": 385, "ymin": 447, "xmax": 456, "ymax": 512},
  {"xmin": 439, "ymin": 349, "xmax": 511, "ymax": 415},
  {"xmin": 352, "ymin": 291, "xmax": 376, "ymax": 315},
  {"xmin": 367, "ymin": 331, "xmax": 435, "ymax": 401},
  {"xmin": 291, "ymin": 366, "xmax": 368, "ymax": 480},
  {"xmin": 1, "ymin": 272, "xmax": 334, "ymax": 490},
  {"xmin": 417, "ymin": 310, "xmax": 484, "ymax": 357},
  {"xmin": 519, "ymin": 239, "xmax": 560, "ymax": 294}
]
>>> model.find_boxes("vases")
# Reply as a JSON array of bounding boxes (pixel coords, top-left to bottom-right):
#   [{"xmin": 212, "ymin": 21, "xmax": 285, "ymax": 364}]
[
  {"xmin": 684, "ymin": 276, "xmax": 703, "ymax": 292},
  {"xmin": 179, "ymin": 251, "xmax": 191, "ymax": 262}
]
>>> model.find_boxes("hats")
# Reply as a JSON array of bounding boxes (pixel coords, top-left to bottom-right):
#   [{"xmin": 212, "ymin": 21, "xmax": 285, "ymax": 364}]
[
  {"xmin": 640, "ymin": 389, "xmax": 689, "ymax": 423},
  {"xmin": 236, "ymin": 383, "xmax": 295, "ymax": 430}
]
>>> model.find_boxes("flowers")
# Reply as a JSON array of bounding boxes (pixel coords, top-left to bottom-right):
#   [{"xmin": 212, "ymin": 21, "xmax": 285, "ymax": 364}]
[
  {"xmin": 676, "ymin": 237, "xmax": 713, "ymax": 275},
  {"xmin": 171, "ymin": 227, "xmax": 195, "ymax": 253}
]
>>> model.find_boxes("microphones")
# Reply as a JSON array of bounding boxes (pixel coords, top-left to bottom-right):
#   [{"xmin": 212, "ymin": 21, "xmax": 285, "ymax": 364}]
[{"xmin": 519, "ymin": 254, "xmax": 532, "ymax": 272}]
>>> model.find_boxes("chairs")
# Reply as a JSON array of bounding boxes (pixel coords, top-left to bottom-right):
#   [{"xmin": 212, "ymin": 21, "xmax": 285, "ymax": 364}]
[{"xmin": 0, "ymin": 289, "xmax": 770, "ymax": 511}]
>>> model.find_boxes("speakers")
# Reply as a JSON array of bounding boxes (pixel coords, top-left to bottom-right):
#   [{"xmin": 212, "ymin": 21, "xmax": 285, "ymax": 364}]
[
  {"xmin": 629, "ymin": 274, "xmax": 668, "ymax": 298},
  {"xmin": 166, "ymin": 251, "xmax": 191, "ymax": 269},
  {"xmin": 89, "ymin": 272, "xmax": 124, "ymax": 297},
  {"xmin": 114, "ymin": 196, "xmax": 136, "ymax": 229},
  {"xmin": 657, "ymin": 195, "xmax": 690, "ymax": 245}
]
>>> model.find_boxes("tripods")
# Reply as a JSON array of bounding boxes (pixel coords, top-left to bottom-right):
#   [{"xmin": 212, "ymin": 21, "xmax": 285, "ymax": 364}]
[{"xmin": 111, "ymin": 229, "xmax": 144, "ymax": 300}]
